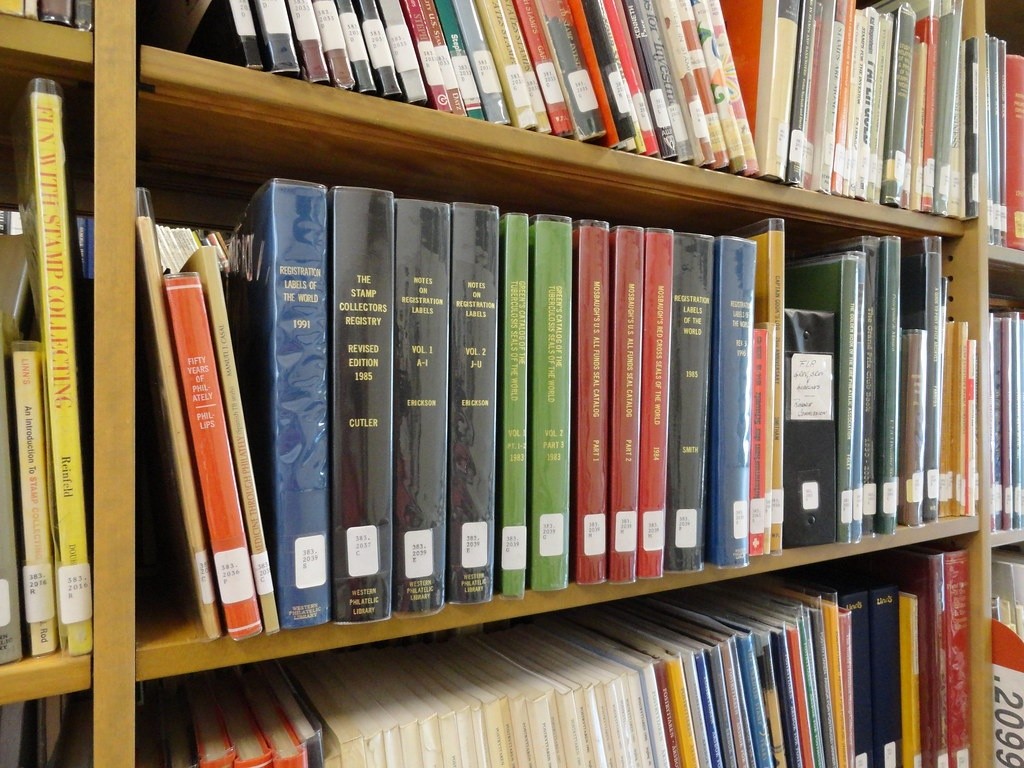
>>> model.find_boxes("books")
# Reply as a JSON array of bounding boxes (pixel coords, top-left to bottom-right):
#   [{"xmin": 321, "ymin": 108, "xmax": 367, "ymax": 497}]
[
  {"xmin": 226, "ymin": 180, "xmax": 332, "ymax": 630},
  {"xmin": 0, "ymin": 1, "xmax": 1023, "ymax": 768}
]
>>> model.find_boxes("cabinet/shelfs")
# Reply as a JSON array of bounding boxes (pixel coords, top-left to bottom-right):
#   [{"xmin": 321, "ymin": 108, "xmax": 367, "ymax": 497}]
[{"xmin": 1, "ymin": 0, "xmax": 1024, "ymax": 768}]
